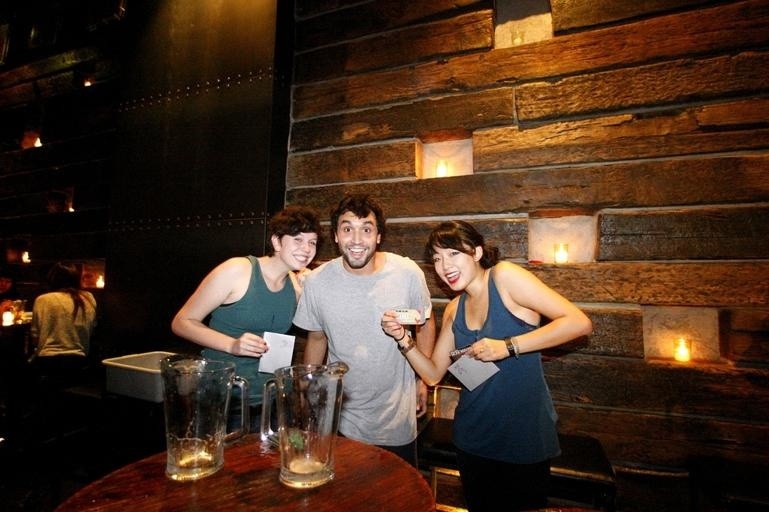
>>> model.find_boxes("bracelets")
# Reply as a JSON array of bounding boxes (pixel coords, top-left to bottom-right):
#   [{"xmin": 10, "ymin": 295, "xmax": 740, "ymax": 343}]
[
  {"xmin": 504, "ymin": 336, "xmax": 520, "ymax": 359},
  {"xmin": 397, "ymin": 335, "xmax": 417, "ymax": 356},
  {"xmin": 395, "ymin": 328, "xmax": 406, "ymax": 342}
]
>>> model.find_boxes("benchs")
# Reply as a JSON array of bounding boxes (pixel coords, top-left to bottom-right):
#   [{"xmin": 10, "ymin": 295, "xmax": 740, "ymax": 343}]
[{"xmin": 407, "ymin": 417, "xmax": 617, "ymax": 512}]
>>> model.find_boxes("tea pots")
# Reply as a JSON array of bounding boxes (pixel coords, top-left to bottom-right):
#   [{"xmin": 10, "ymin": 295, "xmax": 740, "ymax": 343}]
[
  {"xmin": 257, "ymin": 362, "xmax": 350, "ymax": 490},
  {"xmin": 156, "ymin": 354, "xmax": 249, "ymax": 484}
]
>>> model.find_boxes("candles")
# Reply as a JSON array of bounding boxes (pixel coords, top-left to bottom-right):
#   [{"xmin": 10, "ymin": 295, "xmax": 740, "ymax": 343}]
[
  {"xmin": 435, "ymin": 159, "xmax": 448, "ymax": 177},
  {"xmin": 673, "ymin": 339, "xmax": 691, "ymax": 362},
  {"xmin": 553, "ymin": 243, "xmax": 569, "ymax": 264}
]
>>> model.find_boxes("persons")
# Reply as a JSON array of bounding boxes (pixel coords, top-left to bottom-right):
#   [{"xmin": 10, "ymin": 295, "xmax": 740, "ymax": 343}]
[
  {"xmin": 380, "ymin": 219, "xmax": 593, "ymax": 511},
  {"xmin": 170, "ymin": 207, "xmax": 323, "ymax": 440},
  {"xmin": 0, "ymin": 258, "xmax": 99, "ymax": 489},
  {"xmin": 291, "ymin": 192, "xmax": 438, "ymax": 470}
]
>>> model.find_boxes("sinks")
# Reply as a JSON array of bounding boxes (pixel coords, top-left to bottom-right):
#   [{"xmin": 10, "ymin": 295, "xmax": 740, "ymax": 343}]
[{"xmin": 102, "ymin": 351, "xmax": 176, "ymax": 401}]
